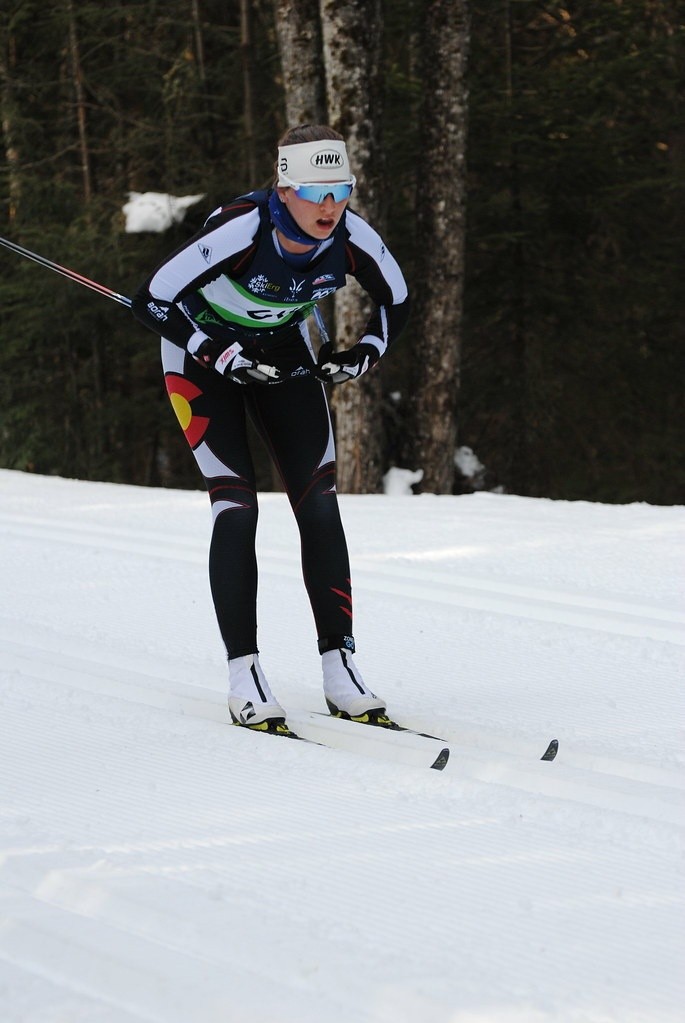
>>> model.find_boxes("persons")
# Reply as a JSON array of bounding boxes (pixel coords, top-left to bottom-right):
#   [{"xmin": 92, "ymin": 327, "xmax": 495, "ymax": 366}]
[{"xmin": 131, "ymin": 123, "xmax": 412, "ymax": 726}]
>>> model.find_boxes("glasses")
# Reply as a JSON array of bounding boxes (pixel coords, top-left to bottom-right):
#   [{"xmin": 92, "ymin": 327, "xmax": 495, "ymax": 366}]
[{"xmin": 292, "ymin": 175, "xmax": 357, "ymax": 204}]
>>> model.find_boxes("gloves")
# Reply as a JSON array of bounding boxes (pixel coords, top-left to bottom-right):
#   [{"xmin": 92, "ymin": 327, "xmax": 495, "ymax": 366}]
[
  {"xmin": 309, "ymin": 341, "xmax": 379, "ymax": 384},
  {"xmin": 195, "ymin": 339, "xmax": 283, "ymax": 387}
]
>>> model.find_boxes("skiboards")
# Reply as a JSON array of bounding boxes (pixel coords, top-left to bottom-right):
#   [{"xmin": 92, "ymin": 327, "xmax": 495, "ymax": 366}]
[{"xmin": 210, "ymin": 706, "xmax": 562, "ymax": 773}]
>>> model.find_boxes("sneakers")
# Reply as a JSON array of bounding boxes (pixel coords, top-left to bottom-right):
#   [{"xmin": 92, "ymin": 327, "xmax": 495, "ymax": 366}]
[
  {"xmin": 321, "ymin": 649, "xmax": 392, "ymax": 725},
  {"xmin": 227, "ymin": 653, "xmax": 292, "ymax": 734}
]
